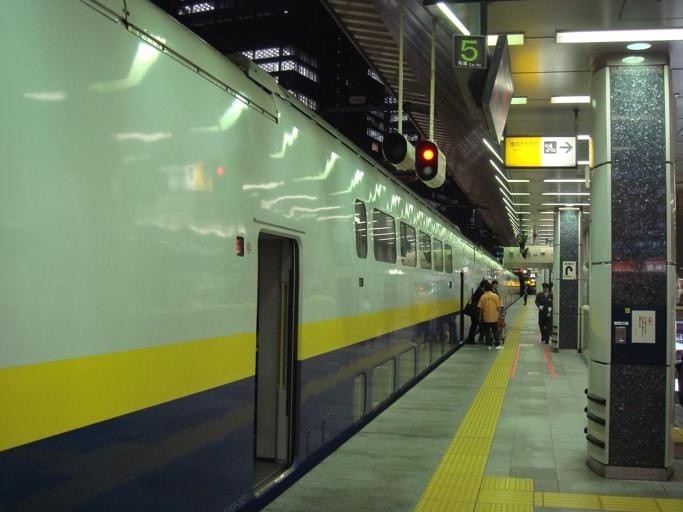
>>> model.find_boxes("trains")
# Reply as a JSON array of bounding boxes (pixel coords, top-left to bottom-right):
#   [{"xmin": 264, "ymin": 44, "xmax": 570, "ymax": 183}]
[{"xmin": 0, "ymin": 0, "xmax": 520, "ymax": 512}]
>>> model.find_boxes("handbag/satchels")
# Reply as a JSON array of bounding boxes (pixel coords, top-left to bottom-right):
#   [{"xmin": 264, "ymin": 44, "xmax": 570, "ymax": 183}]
[{"xmin": 465, "ymin": 302, "xmax": 477, "ymax": 317}]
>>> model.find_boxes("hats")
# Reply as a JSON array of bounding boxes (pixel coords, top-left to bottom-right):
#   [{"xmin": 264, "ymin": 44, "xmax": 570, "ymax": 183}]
[{"xmin": 542, "ymin": 283, "xmax": 549, "ymax": 288}]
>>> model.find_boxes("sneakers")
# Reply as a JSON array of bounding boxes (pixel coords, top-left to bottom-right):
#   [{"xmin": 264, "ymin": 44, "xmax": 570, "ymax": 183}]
[
  {"xmin": 495, "ymin": 344, "xmax": 504, "ymax": 350},
  {"xmin": 466, "ymin": 340, "xmax": 487, "ymax": 345},
  {"xmin": 488, "ymin": 345, "xmax": 492, "ymax": 350}
]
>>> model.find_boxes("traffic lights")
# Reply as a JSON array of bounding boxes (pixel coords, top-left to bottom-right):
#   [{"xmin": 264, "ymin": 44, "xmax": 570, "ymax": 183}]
[{"xmin": 382, "ymin": 132, "xmax": 447, "ymax": 189}]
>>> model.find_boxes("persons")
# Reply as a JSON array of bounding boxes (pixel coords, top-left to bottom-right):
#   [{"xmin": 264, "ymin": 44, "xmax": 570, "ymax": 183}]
[
  {"xmin": 535, "ymin": 283, "xmax": 553, "ymax": 344},
  {"xmin": 523, "ymin": 285, "xmax": 528, "ymax": 305},
  {"xmin": 466, "ymin": 279, "xmax": 505, "ymax": 349}
]
